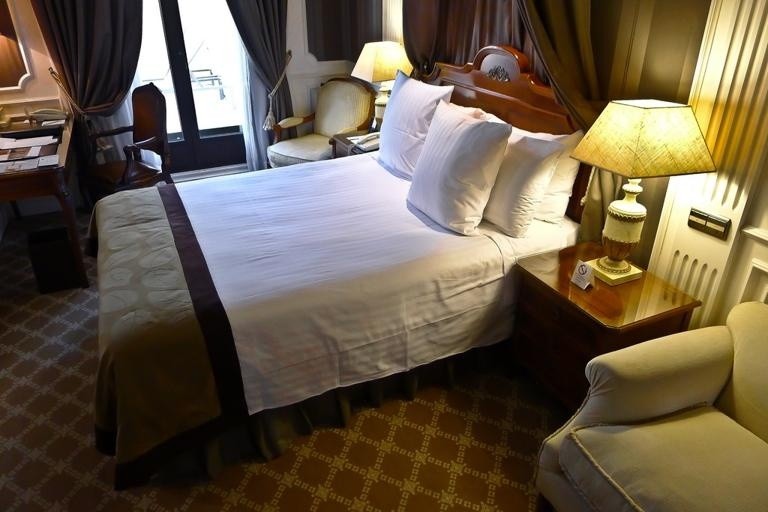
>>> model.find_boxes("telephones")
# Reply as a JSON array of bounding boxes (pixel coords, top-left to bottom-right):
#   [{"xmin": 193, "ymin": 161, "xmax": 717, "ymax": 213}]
[
  {"xmin": 30, "ymin": 109, "xmax": 67, "ymax": 121},
  {"xmin": 356, "ymin": 132, "xmax": 380, "ymax": 151}
]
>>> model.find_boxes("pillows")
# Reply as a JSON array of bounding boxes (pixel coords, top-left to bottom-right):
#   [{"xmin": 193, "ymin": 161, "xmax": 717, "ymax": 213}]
[
  {"xmin": 374, "ymin": 68, "xmax": 456, "ymax": 180},
  {"xmin": 402, "ymin": 95, "xmax": 513, "ymax": 238},
  {"xmin": 502, "ymin": 119, "xmax": 587, "ymax": 225},
  {"xmin": 474, "ymin": 110, "xmax": 567, "ymax": 240}
]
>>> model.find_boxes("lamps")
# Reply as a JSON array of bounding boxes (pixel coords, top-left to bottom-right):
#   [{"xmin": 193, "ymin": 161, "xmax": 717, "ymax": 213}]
[
  {"xmin": 261, "ymin": 50, "xmax": 292, "ymax": 133},
  {"xmin": 567, "ymin": 97, "xmax": 718, "ymax": 289},
  {"xmin": 346, "ymin": 40, "xmax": 416, "ymax": 134}
]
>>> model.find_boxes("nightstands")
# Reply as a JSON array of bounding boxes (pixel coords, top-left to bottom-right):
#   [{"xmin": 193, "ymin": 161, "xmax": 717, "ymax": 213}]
[
  {"xmin": 510, "ymin": 238, "xmax": 707, "ymax": 422},
  {"xmin": 330, "ymin": 124, "xmax": 380, "ymax": 158}
]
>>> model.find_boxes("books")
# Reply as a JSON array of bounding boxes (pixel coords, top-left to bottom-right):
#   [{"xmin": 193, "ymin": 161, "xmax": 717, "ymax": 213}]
[{"xmin": 0, "ymin": 136, "xmax": 59, "ymax": 173}]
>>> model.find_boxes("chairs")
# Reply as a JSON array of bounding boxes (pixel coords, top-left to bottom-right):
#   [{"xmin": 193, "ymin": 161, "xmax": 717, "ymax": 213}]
[
  {"xmin": 528, "ymin": 299, "xmax": 767, "ymax": 510},
  {"xmin": 262, "ymin": 74, "xmax": 378, "ymax": 170},
  {"xmin": 81, "ymin": 81, "xmax": 170, "ymax": 204}
]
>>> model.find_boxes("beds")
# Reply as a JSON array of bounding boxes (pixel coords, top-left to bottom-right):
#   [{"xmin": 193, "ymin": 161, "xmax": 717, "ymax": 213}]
[{"xmin": 75, "ymin": 39, "xmax": 606, "ymax": 491}]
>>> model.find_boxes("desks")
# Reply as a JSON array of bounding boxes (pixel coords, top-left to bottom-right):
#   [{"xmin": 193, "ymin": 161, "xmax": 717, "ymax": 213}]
[{"xmin": 0, "ymin": 110, "xmax": 101, "ymax": 290}]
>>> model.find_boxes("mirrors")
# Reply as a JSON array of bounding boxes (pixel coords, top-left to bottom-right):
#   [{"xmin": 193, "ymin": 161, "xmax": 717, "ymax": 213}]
[{"xmin": 0, "ymin": 0, "xmax": 35, "ymax": 96}]
